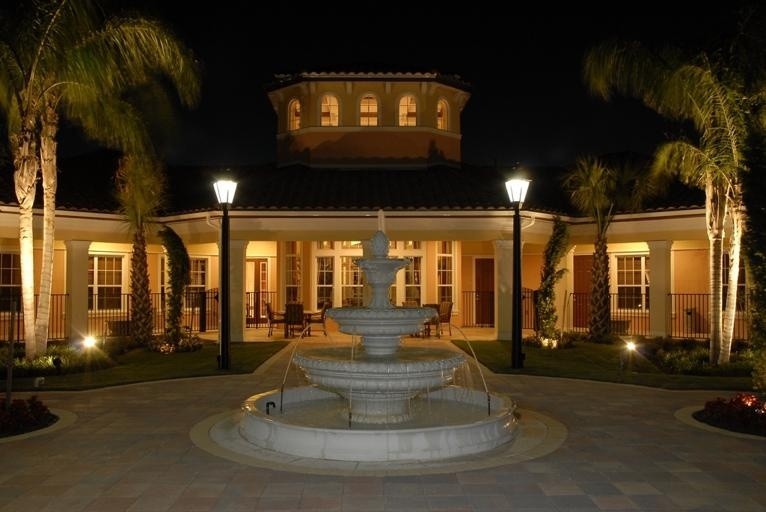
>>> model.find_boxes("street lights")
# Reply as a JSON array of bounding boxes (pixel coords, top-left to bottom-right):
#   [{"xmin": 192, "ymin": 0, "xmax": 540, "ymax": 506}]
[
  {"xmin": 208, "ymin": 166, "xmax": 243, "ymax": 373},
  {"xmin": 499, "ymin": 159, "xmax": 536, "ymax": 365}
]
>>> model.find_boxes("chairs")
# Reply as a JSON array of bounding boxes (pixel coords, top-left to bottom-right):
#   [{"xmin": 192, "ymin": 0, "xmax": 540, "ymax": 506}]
[
  {"xmin": 402, "ymin": 301, "xmax": 453, "ymax": 338},
  {"xmin": 265, "ymin": 302, "xmax": 328, "ymax": 339}
]
